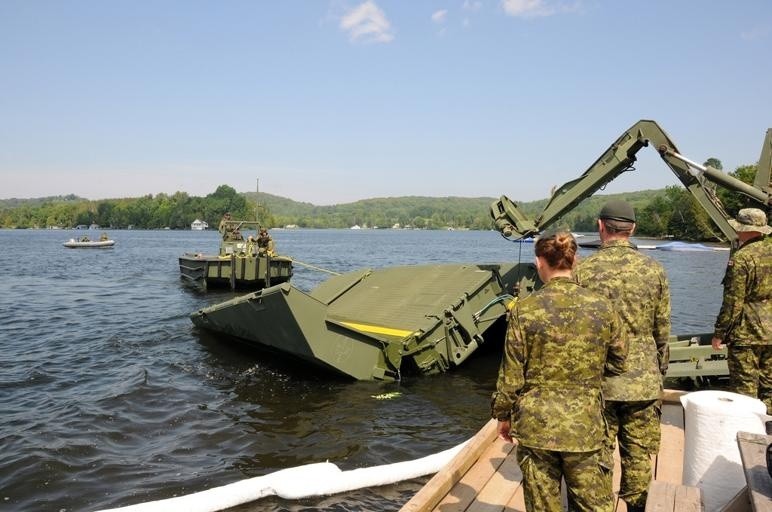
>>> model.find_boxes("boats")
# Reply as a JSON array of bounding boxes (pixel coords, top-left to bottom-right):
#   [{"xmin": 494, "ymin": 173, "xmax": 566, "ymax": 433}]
[
  {"xmin": 62, "ymin": 239, "xmax": 115, "ymax": 248},
  {"xmin": 656, "ymin": 241, "xmax": 715, "ymax": 252},
  {"xmin": 570, "ymin": 233, "xmax": 584, "ymax": 239},
  {"xmin": 575, "ymin": 240, "xmax": 603, "ymax": 249},
  {"xmin": 513, "ymin": 230, "xmax": 546, "ymax": 242},
  {"xmin": 178, "ymin": 178, "xmax": 294, "ymax": 296}
]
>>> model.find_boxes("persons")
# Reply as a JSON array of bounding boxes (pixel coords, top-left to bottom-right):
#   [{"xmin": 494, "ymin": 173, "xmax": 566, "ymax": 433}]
[
  {"xmin": 570, "ymin": 204, "xmax": 671, "ymax": 512},
  {"xmin": 219, "ymin": 212, "xmax": 236, "ymax": 242},
  {"xmin": 100, "ymin": 232, "xmax": 107, "ymax": 241},
  {"xmin": 494, "ymin": 232, "xmax": 630, "ymax": 512},
  {"xmin": 712, "ymin": 208, "xmax": 768, "ymax": 402},
  {"xmin": 81, "ymin": 235, "xmax": 90, "ymax": 242},
  {"xmin": 250, "ymin": 228, "xmax": 272, "ymax": 255}
]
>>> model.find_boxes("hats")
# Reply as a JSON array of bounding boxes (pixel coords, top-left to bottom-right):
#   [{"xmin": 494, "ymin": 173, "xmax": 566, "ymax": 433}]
[
  {"xmin": 599, "ymin": 201, "xmax": 636, "ymax": 223},
  {"xmin": 727, "ymin": 208, "xmax": 772, "ymax": 235}
]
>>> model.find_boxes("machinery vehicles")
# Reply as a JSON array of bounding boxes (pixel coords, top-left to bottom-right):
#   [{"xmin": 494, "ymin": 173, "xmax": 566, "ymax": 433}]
[{"xmin": 489, "ymin": 118, "xmax": 772, "ymax": 389}]
